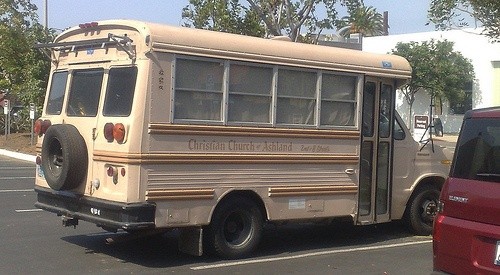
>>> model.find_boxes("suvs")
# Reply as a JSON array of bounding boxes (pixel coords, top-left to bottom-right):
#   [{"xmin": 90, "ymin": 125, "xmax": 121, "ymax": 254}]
[{"xmin": 432, "ymin": 106, "xmax": 500, "ymax": 275}]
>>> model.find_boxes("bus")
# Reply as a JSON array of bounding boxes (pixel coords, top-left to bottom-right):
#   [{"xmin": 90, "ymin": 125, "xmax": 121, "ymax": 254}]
[{"xmin": 32, "ymin": 20, "xmax": 455, "ymax": 259}]
